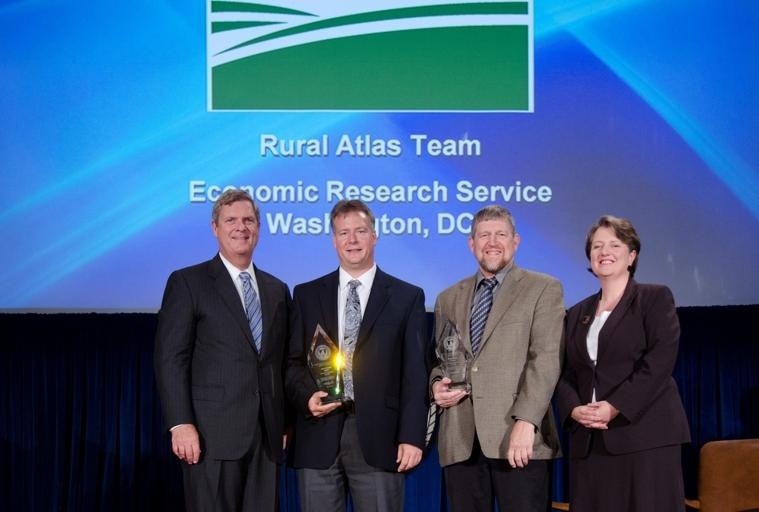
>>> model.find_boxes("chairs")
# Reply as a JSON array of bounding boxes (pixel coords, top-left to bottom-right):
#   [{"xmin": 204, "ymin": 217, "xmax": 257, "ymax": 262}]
[
  {"xmin": 685, "ymin": 437, "xmax": 758, "ymax": 511},
  {"xmin": 552, "ymin": 501, "xmax": 570, "ymax": 512}
]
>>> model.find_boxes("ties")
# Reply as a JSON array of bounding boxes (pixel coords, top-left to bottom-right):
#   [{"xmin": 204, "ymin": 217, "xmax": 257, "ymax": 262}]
[
  {"xmin": 239, "ymin": 272, "xmax": 262, "ymax": 355},
  {"xmin": 470, "ymin": 277, "xmax": 498, "ymax": 356},
  {"xmin": 342, "ymin": 279, "xmax": 362, "ymax": 402}
]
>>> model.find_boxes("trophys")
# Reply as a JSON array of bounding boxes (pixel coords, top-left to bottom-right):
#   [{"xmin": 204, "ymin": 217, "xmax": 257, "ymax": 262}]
[{"xmin": 305, "ymin": 321, "xmax": 353, "ymax": 407}]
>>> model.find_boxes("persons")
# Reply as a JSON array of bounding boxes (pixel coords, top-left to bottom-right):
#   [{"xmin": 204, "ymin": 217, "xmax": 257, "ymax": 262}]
[
  {"xmin": 544, "ymin": 212, "xmax": 693, "ymax": 511},
  {"xmin": 426, "ymin": 204, "xmax": 570, "ymax": 510},
  {"xmin": 151, "ymin": 187, "xmax": 294, "ymax": 511},
  {"xmin": 271, "ymin": 197, "xmax": 433, "ymax": 512}
]
